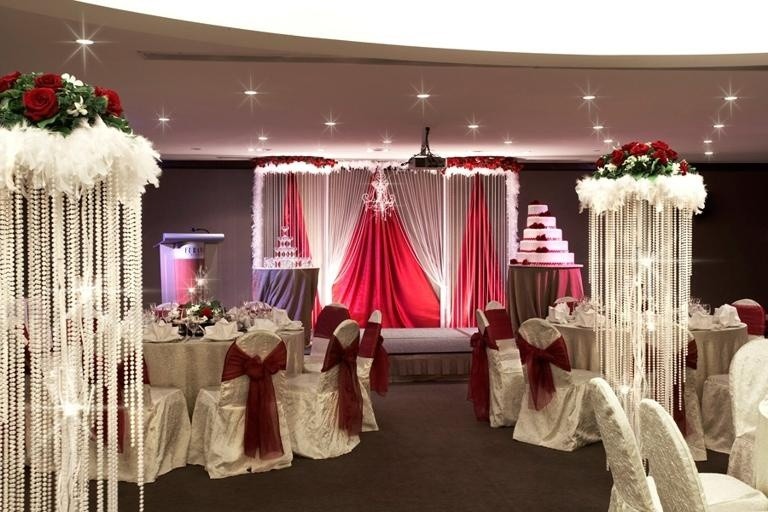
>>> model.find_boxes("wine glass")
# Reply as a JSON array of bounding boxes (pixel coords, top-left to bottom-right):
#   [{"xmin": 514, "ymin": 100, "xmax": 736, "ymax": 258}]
[
  {"xmin": 141, "ymin": 300, "xmax": 281, "ymax": 334},
  {"xmin": 572, "ymin": 292, "xmax": 712, "ymax": 330},
  {"xmin": 260, "ymin": 226, "xmax": 313, "ymax": 269}
]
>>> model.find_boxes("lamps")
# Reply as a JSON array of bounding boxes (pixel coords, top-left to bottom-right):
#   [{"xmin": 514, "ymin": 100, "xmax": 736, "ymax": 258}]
[{"xmin": 358, "ymin": 164, "xmax": 398, "ymax": 220}]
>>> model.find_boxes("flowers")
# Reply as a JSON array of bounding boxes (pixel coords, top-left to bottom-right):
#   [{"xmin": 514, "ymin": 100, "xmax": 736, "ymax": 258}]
[
  {"xmin": 591, "ymin": 139, "xmax": 696, "ymax": 180},
  {"xmin": 0, "ymin": 69, "xmax": 132, "ymax": 134}
]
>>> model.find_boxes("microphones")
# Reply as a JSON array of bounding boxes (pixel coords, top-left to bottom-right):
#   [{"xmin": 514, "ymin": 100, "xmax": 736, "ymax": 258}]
[{"xmin": 191, "ymin": 227, "xmax": 209, "ymax": 233}]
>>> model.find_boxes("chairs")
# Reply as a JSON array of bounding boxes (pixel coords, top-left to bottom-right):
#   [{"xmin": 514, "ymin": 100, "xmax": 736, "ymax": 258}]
[
  {"xmin": 8, "ymin": 290, "xmax": 396, "ymax": 488},
  {"xmin": 588, "ymin": 378, "xmax": 665, "ymax": 511},
  {"xmin": 637, "ymin": 398, "xmax": 768, "ymax": 511},
  {"xmin": 466, "ymin": 294, "xmax": 768, "ymax": 460},
  {"xmin": 726, "ymin": 337, "xmax": 768, "ymax": 488}
]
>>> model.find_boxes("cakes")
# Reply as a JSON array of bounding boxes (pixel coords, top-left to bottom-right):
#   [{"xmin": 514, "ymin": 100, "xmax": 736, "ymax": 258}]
[{"xmin": 514, "ymin": 199, "xmax": 575, "ymax": 264}]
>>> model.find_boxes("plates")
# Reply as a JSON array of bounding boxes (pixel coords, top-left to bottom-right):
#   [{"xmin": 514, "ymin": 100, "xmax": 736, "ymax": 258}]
[
  {"xmin": 283, "ymin": 325, "xmax": 302, "ymax": 331},
  {"xmin": 205, "ymin": 333, "xmax": 243, "ymax": 342},
  {"xmin": 143, "ymin": 336, "xmax": 179, "ymax": 343}
]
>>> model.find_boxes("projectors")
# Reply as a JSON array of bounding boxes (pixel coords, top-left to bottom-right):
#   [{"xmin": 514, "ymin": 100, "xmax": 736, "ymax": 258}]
[{"xmin": 409, "ymin": 153, "xmax": 448, "ymax": 172}]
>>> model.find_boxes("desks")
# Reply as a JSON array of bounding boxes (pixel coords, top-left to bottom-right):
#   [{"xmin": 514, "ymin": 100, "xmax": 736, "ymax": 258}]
[
  {"xmin": 253, "ymin": 268, "xmax": 317, "ymax": 345},
  {"xmin": 506, "ymin": 264, "xmax": 584, "ymax": 331}
]
